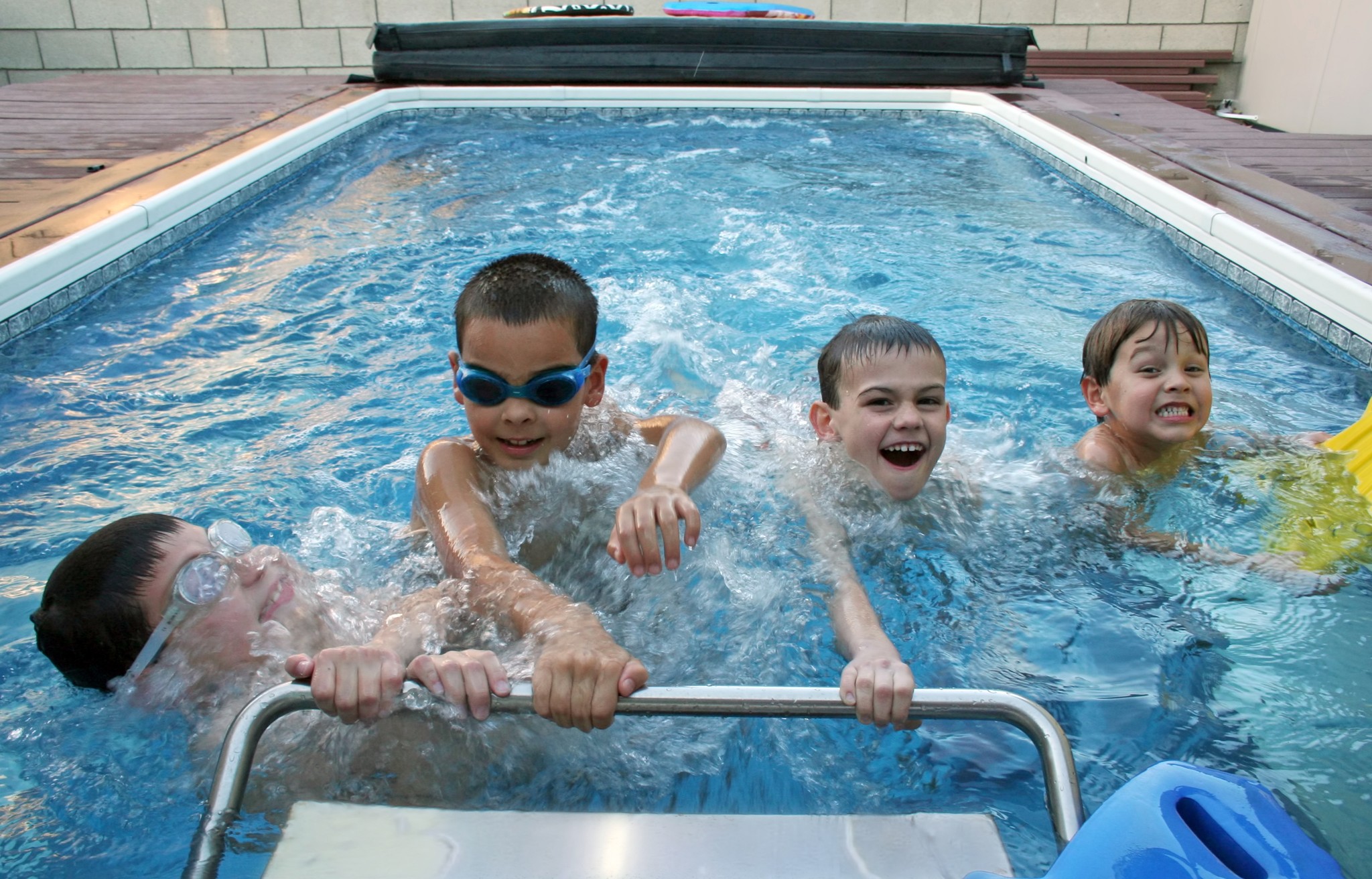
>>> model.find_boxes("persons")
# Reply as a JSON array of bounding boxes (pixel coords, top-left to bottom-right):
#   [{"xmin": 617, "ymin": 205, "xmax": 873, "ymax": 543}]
[
  {"xmin": 1071, "ymin": 298, "xmax": 1348, "ymax": 597},
  {"xmin": 29, "ymin": 514, "xmax": 512, "ymax": 730},
  {"xmin": 780, "ymin": 315, "xmax": 952, "ymax": 731},
  {"xmin": 401, "ymin": 254, "xmax": 728, "ymax": 734}
]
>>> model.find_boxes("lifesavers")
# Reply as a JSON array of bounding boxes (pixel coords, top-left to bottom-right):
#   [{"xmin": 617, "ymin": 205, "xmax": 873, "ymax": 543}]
[
  {"xmin": 502, "ymin": 5, "xmax": 633, "ymax": 18},
  {"xmin": 662, "ymin": 2, "xmax": 815, "ymax": 19}
]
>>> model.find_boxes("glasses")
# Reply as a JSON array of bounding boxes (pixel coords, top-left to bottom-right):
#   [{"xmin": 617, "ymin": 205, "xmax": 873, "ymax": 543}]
[
  {"xmin": 456, "ymin": 363, "xmax": 592, "ymax": 408},
  {"xmin": 162, "ymin": 518, "xmax": 252, "ymax": 627}
]
